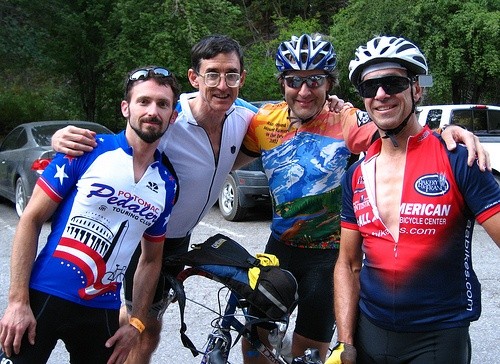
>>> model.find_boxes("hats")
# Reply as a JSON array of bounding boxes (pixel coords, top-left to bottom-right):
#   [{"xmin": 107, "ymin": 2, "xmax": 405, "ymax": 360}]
[{"xmin": 246, "ymin": 268, "xmax": 297, "ymax": 320}]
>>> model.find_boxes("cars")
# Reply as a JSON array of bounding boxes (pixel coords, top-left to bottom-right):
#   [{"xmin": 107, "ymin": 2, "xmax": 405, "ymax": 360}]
[
  {"xmin": 217, "ymin": 100, "xmax": 285, "ymax": 222},
  {"xmin": 0, "ymin": 120, "xmax": 116, "ymax": 223}
]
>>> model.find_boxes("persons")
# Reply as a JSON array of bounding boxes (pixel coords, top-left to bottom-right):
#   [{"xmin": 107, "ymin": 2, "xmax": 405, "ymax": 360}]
[
  {"xmin": 230, "ymin": 35, "xmax": 500, "ymax": 364},
  {"xmin": 51, "ymin": 35, "xmax": 353, "ymax": 364},
  {"xmin": 0, "ymin": 65, "xmax": 180, "ymax": 364}
]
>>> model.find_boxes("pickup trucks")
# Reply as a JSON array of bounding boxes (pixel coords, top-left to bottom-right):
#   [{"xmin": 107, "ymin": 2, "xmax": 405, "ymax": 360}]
[{"xmin": 415, "ymin": 104, "xmax": 500, "ymax": 186}]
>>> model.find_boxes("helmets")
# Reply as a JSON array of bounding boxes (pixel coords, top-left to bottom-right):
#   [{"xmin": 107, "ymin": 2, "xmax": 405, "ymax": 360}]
[
  {"xmin": 348, "ymin": 36, "xmax": 428, "ymax": 89},
  {"xmin": 274, "ymin": 34, "xmax": 338, "ymax": 75}
]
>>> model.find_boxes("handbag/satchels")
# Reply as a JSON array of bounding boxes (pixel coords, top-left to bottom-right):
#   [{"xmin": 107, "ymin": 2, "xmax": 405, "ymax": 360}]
[{"xmin": 175, "ymin": 233, "xmax": 299, "ymax": 330}]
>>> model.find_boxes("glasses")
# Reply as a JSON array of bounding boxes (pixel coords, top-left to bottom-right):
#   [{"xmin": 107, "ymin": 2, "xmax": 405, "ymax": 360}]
[
  {"xmin": 358, "ymin": 75, "xmax": 417, "ymax": 98},
  {"xmin": 282, "ymin": 74, "xmax": 328, "ymax": 88},
  {"xmin": 125, "ymin": 67, "xmax": 175, "ymax": 100},
  {"xmin": 195, "ymin": 70, "xmax": 242, "ymax": 89}
]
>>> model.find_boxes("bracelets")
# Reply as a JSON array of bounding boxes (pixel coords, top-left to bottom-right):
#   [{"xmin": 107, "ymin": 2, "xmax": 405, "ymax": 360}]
[
  {"xmin": 130, "ymin": 317, "xmax": 145, "ymax": 334},
  {"xmin": 441, "ymin": 124, "xmax": 449, "ymax": 131}
]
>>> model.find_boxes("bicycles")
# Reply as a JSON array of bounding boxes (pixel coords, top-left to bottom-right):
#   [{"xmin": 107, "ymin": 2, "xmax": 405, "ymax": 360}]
[{"xmin": 160, "ymin": 256, "xmax": 325, "ymax": 364}]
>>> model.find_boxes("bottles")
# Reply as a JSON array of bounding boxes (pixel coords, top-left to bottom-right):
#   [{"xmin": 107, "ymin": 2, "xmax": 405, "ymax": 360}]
[{"xmin": 268, "ymin": 329, "xmax": 293, "ymax": 364}]
